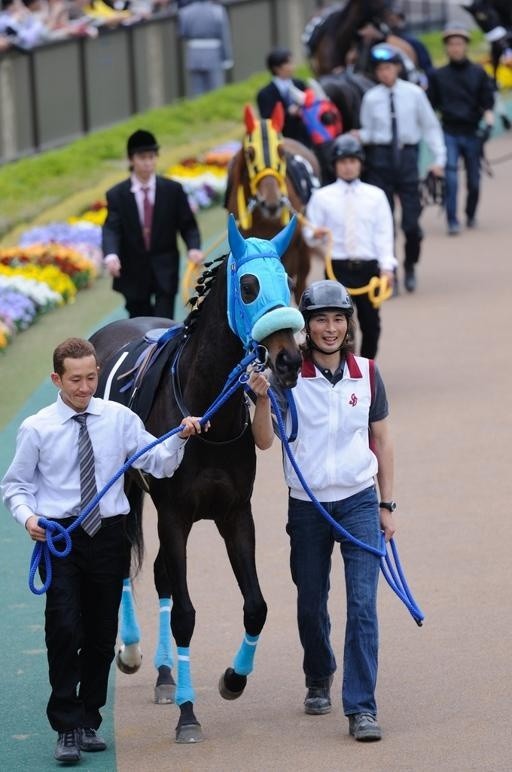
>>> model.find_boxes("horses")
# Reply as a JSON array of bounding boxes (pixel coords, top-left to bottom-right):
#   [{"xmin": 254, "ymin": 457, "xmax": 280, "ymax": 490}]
[
  {"xmin": 302, "ymin": 1, "xmax": 433, "ymax": 77},
  {"xmin": 76, "ymin": 209, "xmax": 308, "ymax": 748},
  {"xmin": 292, "ymin": 64, "xmax": 382, "ymax": 179},
  {"xmin": 220, "ymin": 95, "xmax": 324, "ymax": 305},
  {"xmin": 460, "ymin": 1, "xmax": 510, "ymax": 69}
]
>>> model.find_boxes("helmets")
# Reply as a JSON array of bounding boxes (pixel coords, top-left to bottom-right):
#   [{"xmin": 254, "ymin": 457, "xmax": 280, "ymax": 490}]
[
  {"xmin": 298, "ymin": 279, "xmax": 355, "ymax": 315},
  {"xmin": 443, "ymin": 21, "xmax": 472, "ymax": 43},
  {"xmin": 330, "ymin": 133, "xmax": 364, "ymax": 158},
  {"xmin": 371, "ymin": 43, "xmax": 402, "ymax": 64}
]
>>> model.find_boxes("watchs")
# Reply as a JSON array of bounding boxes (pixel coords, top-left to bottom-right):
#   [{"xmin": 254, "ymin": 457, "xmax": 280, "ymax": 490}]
[{"xmin": 378, "ymin": 500, "xmax": 398, "ymax": 512}]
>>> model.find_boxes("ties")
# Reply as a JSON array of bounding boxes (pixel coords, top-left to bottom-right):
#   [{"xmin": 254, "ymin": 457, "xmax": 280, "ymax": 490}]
[
  {"xmin": 344, "ymin": 186, "xmax": 357, "ymax": 259},
  {"xmin": 71, "ymin": 411, "xmax": 103, "ymax": 539},
  {"xmin": 139, "ymin": 187, "xmax": 154, "ymax": 251}
]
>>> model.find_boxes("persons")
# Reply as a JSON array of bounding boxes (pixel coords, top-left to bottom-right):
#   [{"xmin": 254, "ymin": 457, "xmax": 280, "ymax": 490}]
[
  {"xmin": 1, "ymin": 338, "xmax": 212, "ymax": 762},
  {"xmin": 256, "ymin": 1, "xmax": 510, "ymax": 290},
  {"xmin": 100, "ymin": 127, "xmax": 210, "ymax": 320},
  {"xmin": 300, "ymin": 131, "xmax": 397, "ymax": 362},
  {"xmin": 0, "ymin": 0, "xmax": 174, "ymax": 59},
  {"xmin": 244, "ymin": 281, "xmax": 400, "ymax": 741}
]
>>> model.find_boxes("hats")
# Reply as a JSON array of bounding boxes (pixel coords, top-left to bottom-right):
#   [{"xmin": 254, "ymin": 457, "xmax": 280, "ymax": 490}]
[{"xmin": 128, "ymin": 129, "xmax": 160, "ymax": 152}]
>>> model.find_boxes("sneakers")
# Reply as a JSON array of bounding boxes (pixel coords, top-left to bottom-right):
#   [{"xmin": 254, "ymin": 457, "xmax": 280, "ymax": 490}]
[
  {"xmin": 467, "ymin": 215, "xmax": 477, "ymax": 231},
  {"xmin": 447, "ymin": 221, "xmax": 461, "ymax": 235},
  {"xmin": 349, "ymin": 712, "xmax": 382, "ymax": 742},
  {"xmin": 53, "ymin": 726, "xmax": 82, "ymax": 762},
  {"xmin": 303, "ymin": 671, "xmax": 335, "ymax": 715},
  {"xmin": 405, "ymin": 267, "xmax": 416, "ymax": 293},
  {"xmin": 79, "ymin": 726, "xmax": 107, "ymax": 753}
]
